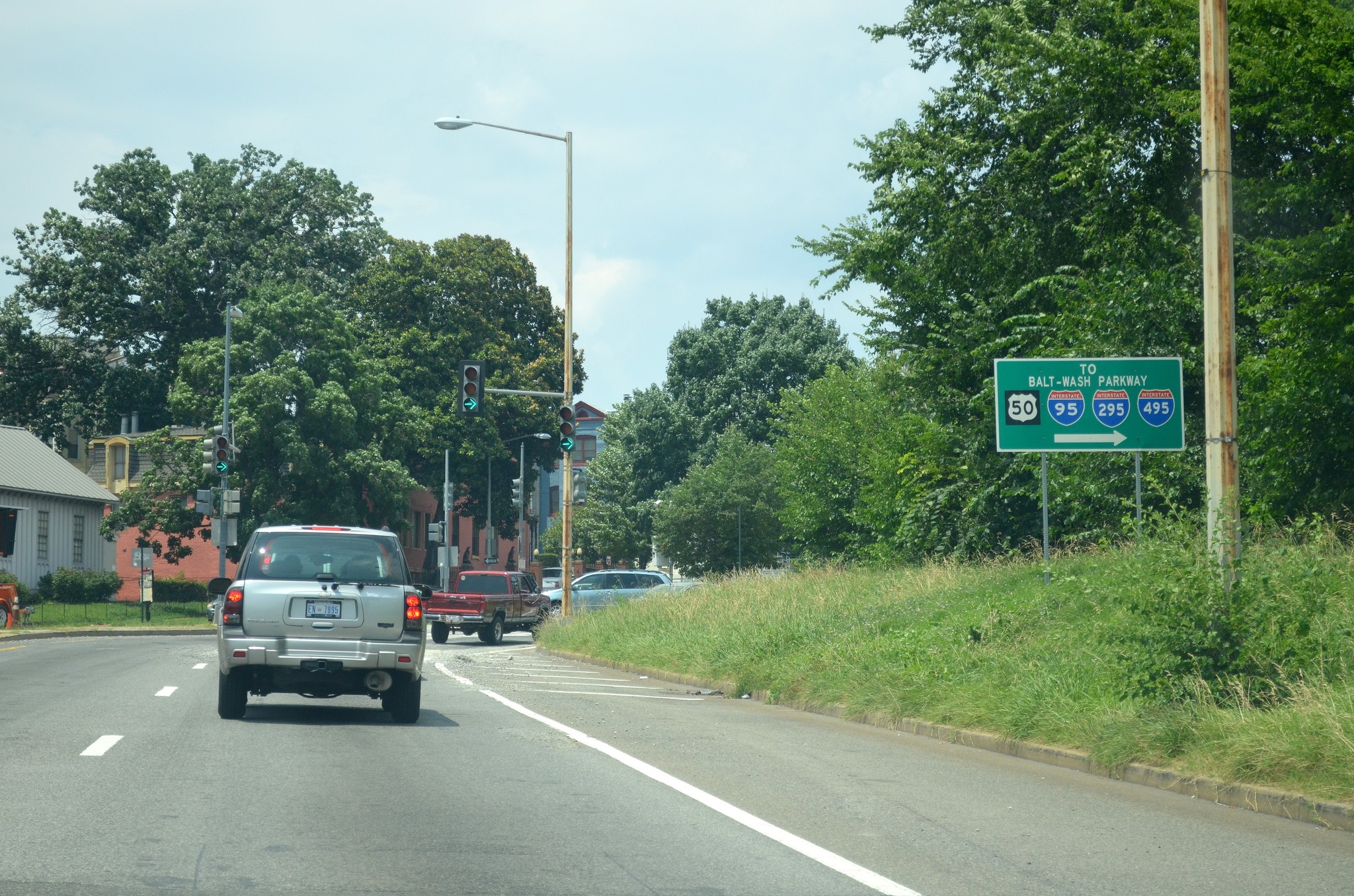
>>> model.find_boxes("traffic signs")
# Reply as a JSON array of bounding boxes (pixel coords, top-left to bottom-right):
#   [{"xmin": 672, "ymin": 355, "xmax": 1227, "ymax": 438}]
[
  {"xmin": 995, "ymin": 356, "xmax": 1186, "ymax": 454},
  {"xmin": 484, "ymin": 558, "xmax": 498, "ymax": 565}
]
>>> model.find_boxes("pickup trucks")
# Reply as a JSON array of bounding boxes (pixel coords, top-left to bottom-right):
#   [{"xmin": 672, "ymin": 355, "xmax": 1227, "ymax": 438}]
[{"xmin": 422, "ymin": 571, "xmax": 551, "ymax": 644}]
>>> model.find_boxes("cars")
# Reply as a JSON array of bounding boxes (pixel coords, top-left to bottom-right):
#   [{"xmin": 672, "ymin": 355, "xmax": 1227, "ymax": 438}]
[
  {"xmin": 207, "ymin": 598, "xmax": 218, "ymax": 622},
  {"xmin": 641, "ymin": 582, "xmax": 710, "ymax": 599},
  {"xmin": 541, "ymin": 567, "xmax": 575, "ymax": 592}
]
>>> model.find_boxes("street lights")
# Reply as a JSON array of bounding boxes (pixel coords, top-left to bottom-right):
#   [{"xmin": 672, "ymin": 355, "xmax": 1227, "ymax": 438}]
[
  {"xmin": 653, "ymin": 500, "xmax": 672, "ymax": 581},
  {"xmin": 482, "ymin": 433, "xmax": 553, "ymax": 573},
  {"xmin": 210, "ymin": 301, "xmax": 243, "ymax": 609},
  {"xmin": 434, "ymin": 117, "xmax": 576, "ymax": 617}
]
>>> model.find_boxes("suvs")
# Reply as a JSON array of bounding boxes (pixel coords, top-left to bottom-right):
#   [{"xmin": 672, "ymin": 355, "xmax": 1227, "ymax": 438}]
[
  {"xmin": 538, "ymin": 570, "xmax": 675, "ymax": 619},
  {"xmin": 206, "ymin": 525, "xmax": 432, "ymax": 724}
]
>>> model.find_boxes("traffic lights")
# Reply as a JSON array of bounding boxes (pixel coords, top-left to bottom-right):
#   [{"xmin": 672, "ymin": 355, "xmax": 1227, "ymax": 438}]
[
  {"xmin": 457, "ymin": 359, "xmax": 485, "ymax": 418},
  {"xmin": 558, "ymin": 405, "xmax": 577, "ymax": 453},
  {"xmin": 212, "ymin": 434, "xmax": 230, "ymax": 474},
  {"xmin": 193, "ymin": 490, "xmax": 215, "ymax": 516},
  {"xmin": 223, "ymin": 489, "xmax": 241, "ymax": 515},
  {"xmin": 509, "ymin": 479, "xmax": 521, "ymax": 508},
  {"xmin": 428, "ymin": 524, "xmax": 441, "ymax": 542},
  {"xmin": 202, "ymin": 438, "xmax": 215, "ymax": 473}
]
